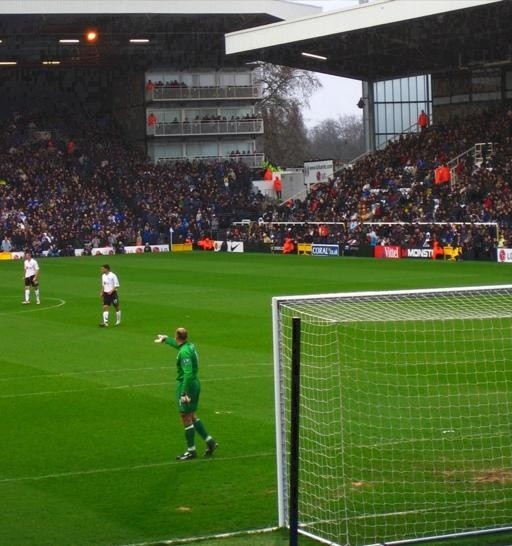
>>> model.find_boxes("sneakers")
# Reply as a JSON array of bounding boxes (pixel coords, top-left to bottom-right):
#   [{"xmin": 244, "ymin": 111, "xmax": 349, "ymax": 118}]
[
  {"xmin": 204, "ymin": 443, "xmax": 219, "ymax": 457},
  {"xmin": 99, "ymin": 322, "xmax": 108, "ymax": 327},
  {"xmin": 22, "ymin": 301, "xmax": 30, "ymax": 305},
  {"xmin": 176, "ymin": 451, "xmax": 197, "ymax": 460},
  {"xmin": 114, "ymin": 321, "xmax": 120, "ymax": 326},
  {"xmin": 36, "ymin": 301, "xmax": 40, "ymax": 304}
]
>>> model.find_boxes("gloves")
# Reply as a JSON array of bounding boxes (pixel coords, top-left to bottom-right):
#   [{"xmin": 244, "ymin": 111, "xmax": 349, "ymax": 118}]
[
  {"xmin": 153, "ymin": 334, "xmax": 168, "ymax": 343},
  {"xmin": 180, "ymin": 395, "xmax": 192, "ymax": 404}
]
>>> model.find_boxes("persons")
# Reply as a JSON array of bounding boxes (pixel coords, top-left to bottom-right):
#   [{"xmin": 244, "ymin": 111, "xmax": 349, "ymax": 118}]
[
  {"xmin": 99, "ymin": 265, "xmax": 121, "ymax": 328},
  {"xmin": 22, "ymin": 248, "xmax": 40, "ymax": 305},
  {"xmin": 156, "ymin": 328, "xmax": 219, "ymax": 461}
]
